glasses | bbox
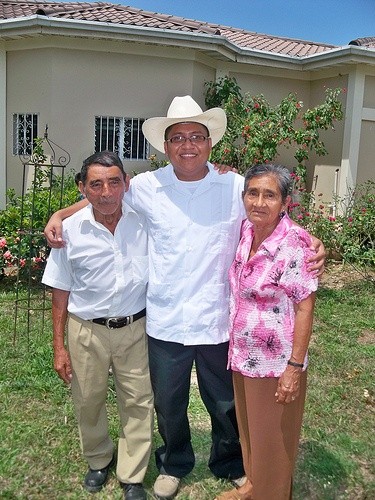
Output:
[166,135,209,144]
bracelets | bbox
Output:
[288,360,304,367]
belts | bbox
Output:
[92,308,148,329]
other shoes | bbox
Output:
[154,473,180,496]
[232,476,248,488]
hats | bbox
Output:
[142,96,228,154]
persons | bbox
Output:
[44,95,326,500]
[216,164,319,500]
[75,172,86,199]
[41,153,155,500]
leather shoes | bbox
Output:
[124,482,147,500]
[85,456,116,492]
